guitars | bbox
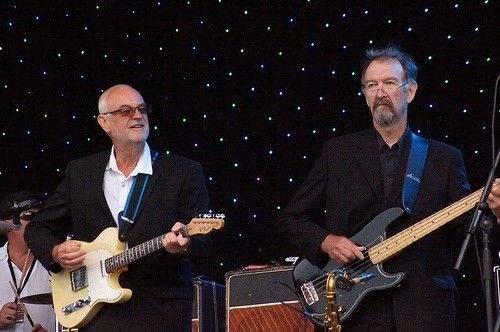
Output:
[293,178,500,326]
[51,213,225,329]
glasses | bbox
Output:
[360,81,408,97]
[103,102,153,117]
[6,211,36,221]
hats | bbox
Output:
[0,189,49,220]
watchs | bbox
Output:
[178,243,190,256]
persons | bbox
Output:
[0,190,56,332]
[277,47,500,332]
[24,84,210,332]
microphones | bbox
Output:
[11,201,22,230]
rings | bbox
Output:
[7,315,11,320]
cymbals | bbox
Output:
[19,292,52,305]
